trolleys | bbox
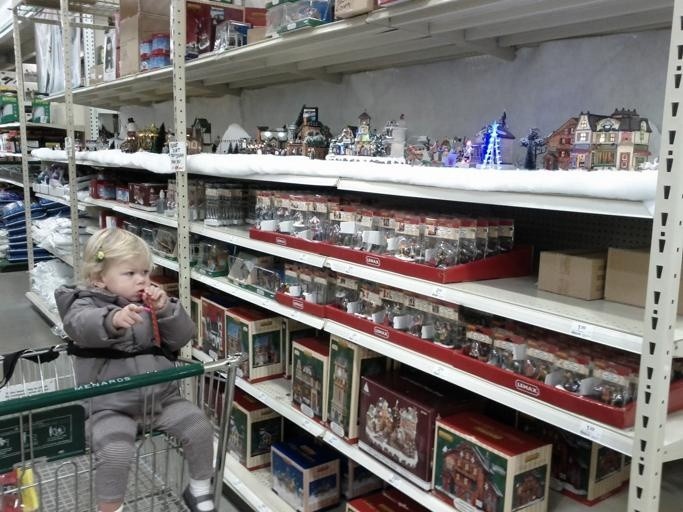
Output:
[0,340,250,511]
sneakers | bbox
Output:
[182,484,217,512]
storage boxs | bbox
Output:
[118,0,170,78]
[291,334,329,426]
[358,371,445,492]
[225,307,283,384]
[105,29,119,82]
[517,410,624,506]
[604,246,682,316]
[538,247,606,301]
[340,455,383,501]
[200,295,233,361]
[270,438,339,511]
[326,334,391,443]
[186,0,245,58]
[432,410,552,512]
[221,387,285,471]
[264,0,332,36]
[280,314,318,378]
[345,492,429,512]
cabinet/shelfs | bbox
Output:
[1,7,105,272]
[11,0,683,512]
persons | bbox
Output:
[53,226,219,512]
